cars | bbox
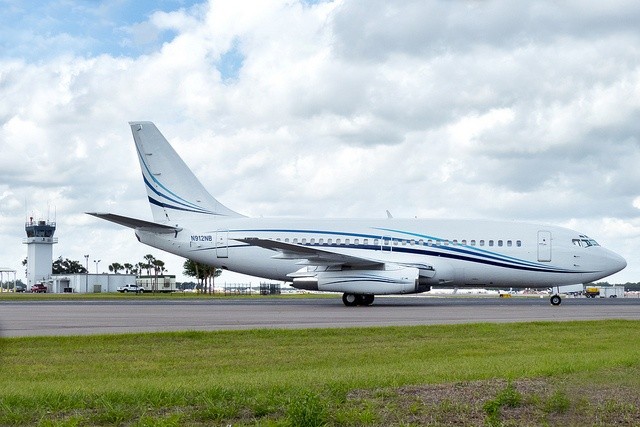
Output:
[11,286,25,293]
[117,284,145,293]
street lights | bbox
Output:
[94,259,101,274]
[85,254,89,292]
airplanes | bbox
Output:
[85,120,627,307]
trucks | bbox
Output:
[547,282,584,296]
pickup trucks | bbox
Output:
[31,283,47,293]
